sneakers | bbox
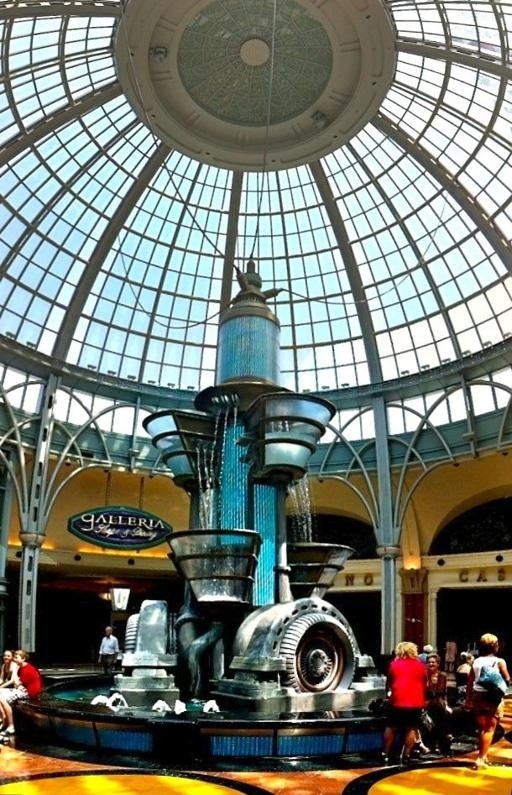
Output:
[471,756,492,770]
[381,744,432,766]
[0,722,15,738]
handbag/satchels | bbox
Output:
[476,665,508,699]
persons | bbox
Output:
[399,632,479,766]
[0,647,19,732]
[98,626,119,675]
[1,650,41,735]
[381,641,425,763]
[464,633,510,768]
